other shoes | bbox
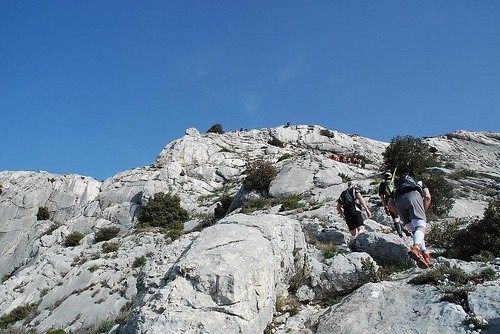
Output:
[403,228,411,236]
[400,235,403,238]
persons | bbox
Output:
[379,172,412,238]
[330,154,365,169]
[387,174,431,269]
[337,181,371,237]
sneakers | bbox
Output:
[422,250,430,263]
[408,247,428,269]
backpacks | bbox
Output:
[342,189,357,208]
[397,175,422,193]
[383,180,396,196]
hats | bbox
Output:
[385,172,392,179]
[348,181,356,185]
